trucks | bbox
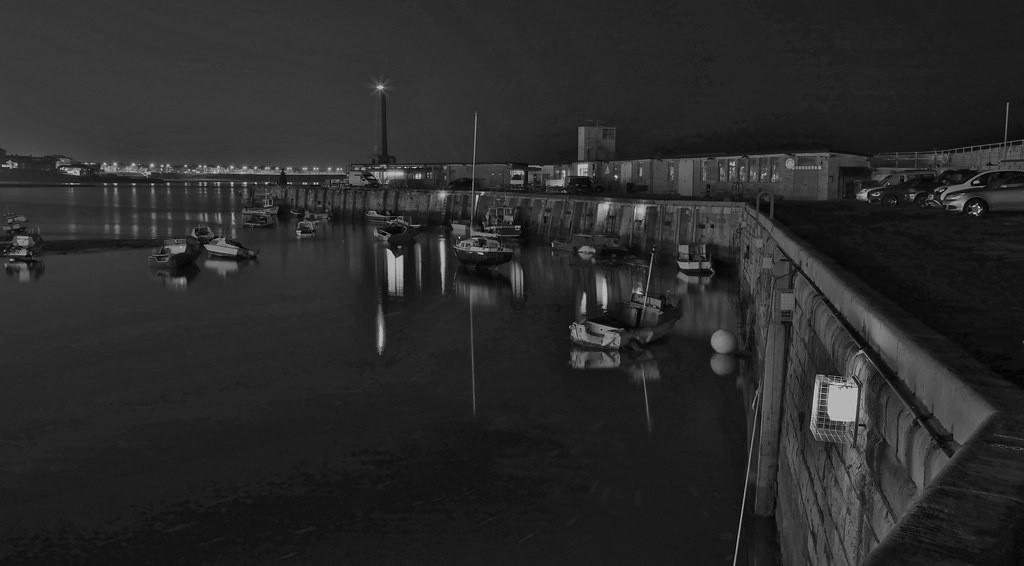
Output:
[349,171,379,188]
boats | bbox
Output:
[480,206,521,236]
[453,232,514,266]
[373,215,428,243]
[677,242,714,278]
[146,238,203,270]
[241,187,279,227]
[569,244,683,349]
[1,224,46,270]
[552,232,627,259]
[191,224,260,259]
[365,210,405,223]
[297,220,316,237]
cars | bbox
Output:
[856,168,1023,209]
[942,173,1024,220]
[564,175,605,194]
